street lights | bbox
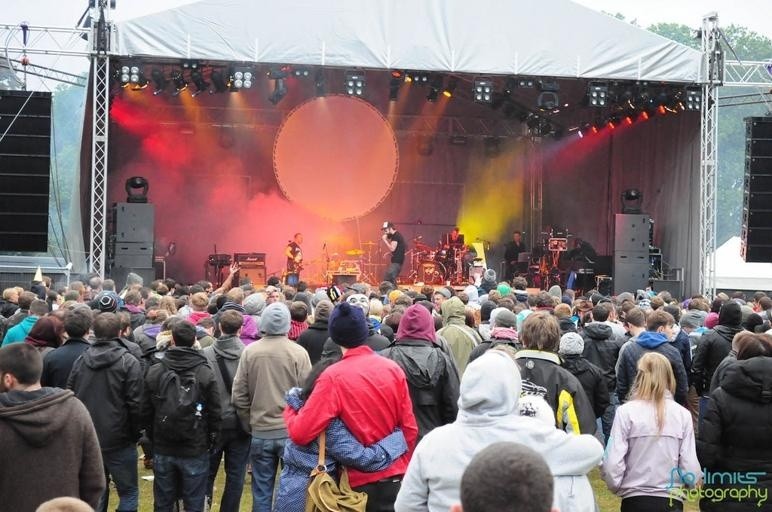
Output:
[304,464,369,512]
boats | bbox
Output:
[111,265,155,294]
[652,279,682,302]
[331,275,357,285]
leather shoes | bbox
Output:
[692,373,705,398]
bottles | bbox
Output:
[704,311,720,330]
[435,287,452,299]
[733,291,747,301]
[518,395,557,426]
[548,285,561,299]
[559,332,584,355]
[380,221,393,231]
[637,299,651,310]
[488,289,501,303]
[714,291,729,302]
[483,268,497,282]
[679,308,702,330]
[496,283,511,298]
[562,296,571,305]
[463,285,478,301]
[480,301,496,322]
[495,309,517,332]
[420,284,434,299]
[575,301,593,312]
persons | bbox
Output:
[447,227,464,244]
[284,233,304,286]
[570,238,598,264]
[504,231,527,273]
[382,221,406,283]
[461,243,477,273]
[0,260,772,512]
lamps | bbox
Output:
[113,58,701,141]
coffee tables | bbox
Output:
[359,243,378,245]
[346,250,366,254]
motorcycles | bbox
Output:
[360,245,380,286]
[407,252,421,280]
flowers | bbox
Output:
[323,241,326,249]
[476,237,489,243]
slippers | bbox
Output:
[152,362,204,450]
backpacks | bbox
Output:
[340,260,361,272]
[417,259,447,285]
